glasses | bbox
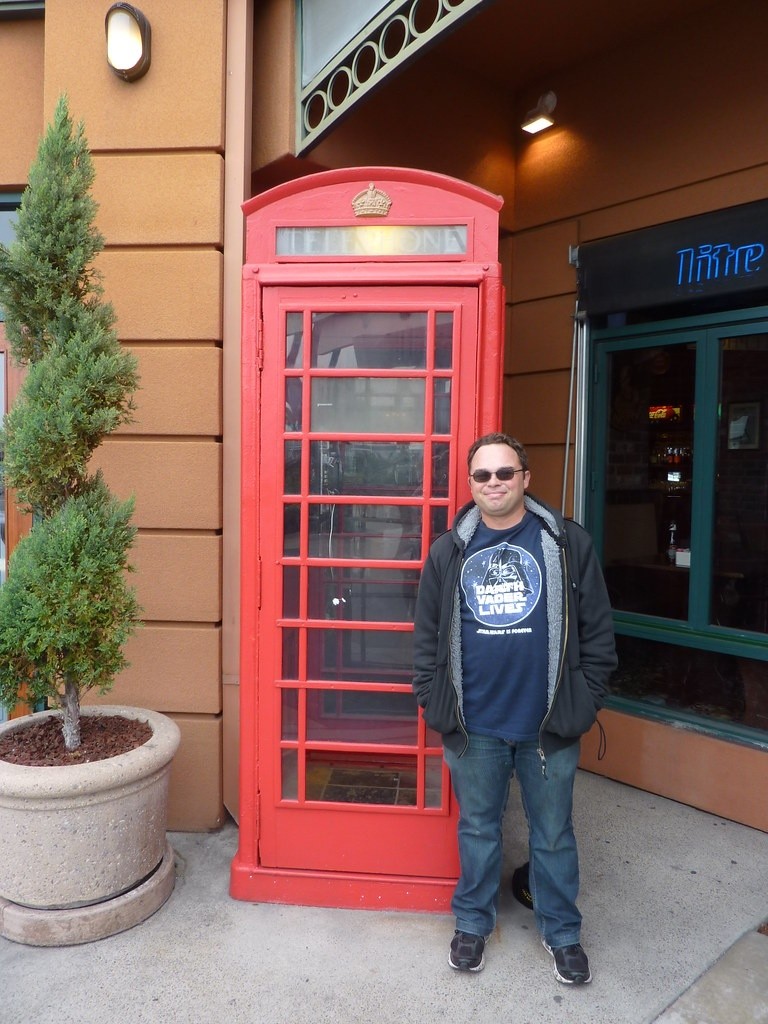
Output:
[469,467,528,482]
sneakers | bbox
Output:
[541,935,592,983]
[448,929,492,972]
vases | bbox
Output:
[726,399,762,452]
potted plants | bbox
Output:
[0,88,183,907]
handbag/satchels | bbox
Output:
[512,860,535,910]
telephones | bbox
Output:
[309,440,341,520]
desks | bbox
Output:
[623,554,768,620]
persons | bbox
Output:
[411,433,619,984]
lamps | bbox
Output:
[520,91,557,135]
[105,1,151,84]
